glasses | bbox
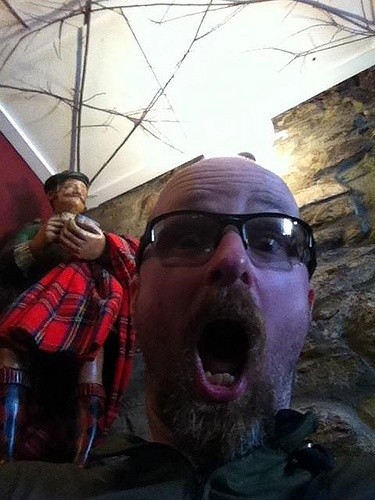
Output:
[134,208,318,281]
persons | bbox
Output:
[0,167,135,471]
[0,157,375,499]
